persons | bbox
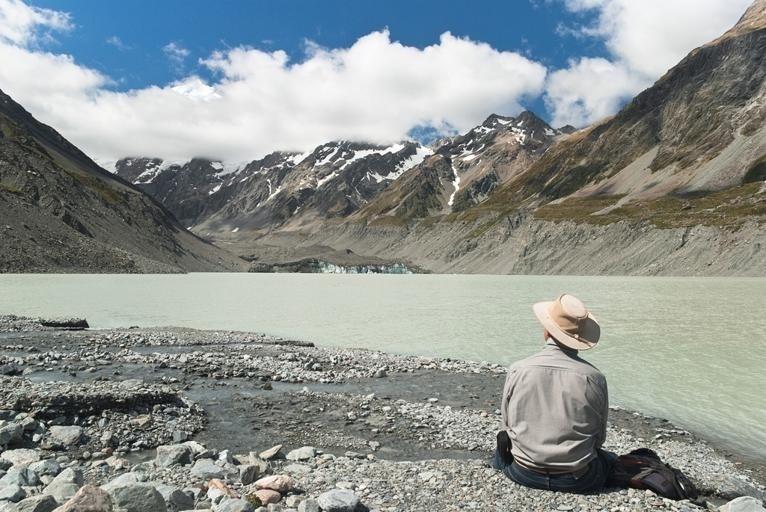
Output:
[495,293,607,490]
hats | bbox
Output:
[533,293,600,350]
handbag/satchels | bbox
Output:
[610,448,697,500]
